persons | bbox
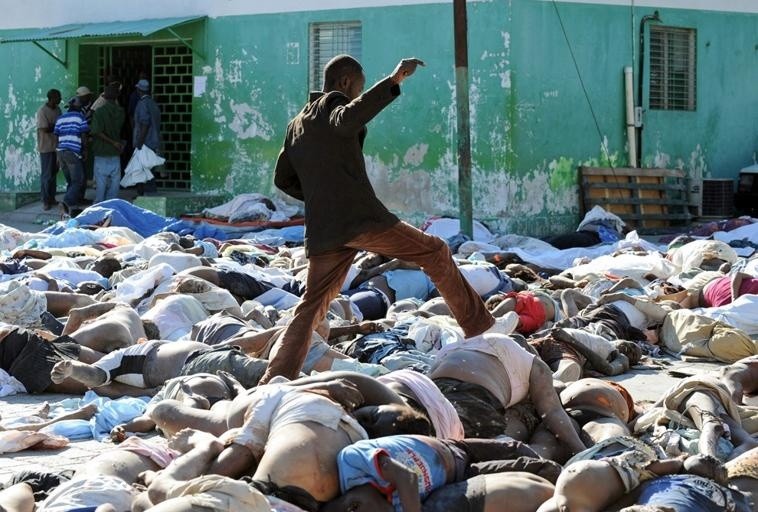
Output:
[0,188,758,511]
[36,69,164,215]
[251,52,519,387]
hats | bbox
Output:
[68,75,150,106]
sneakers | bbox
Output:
[478,309,519,338]
[39,196,89,216]
